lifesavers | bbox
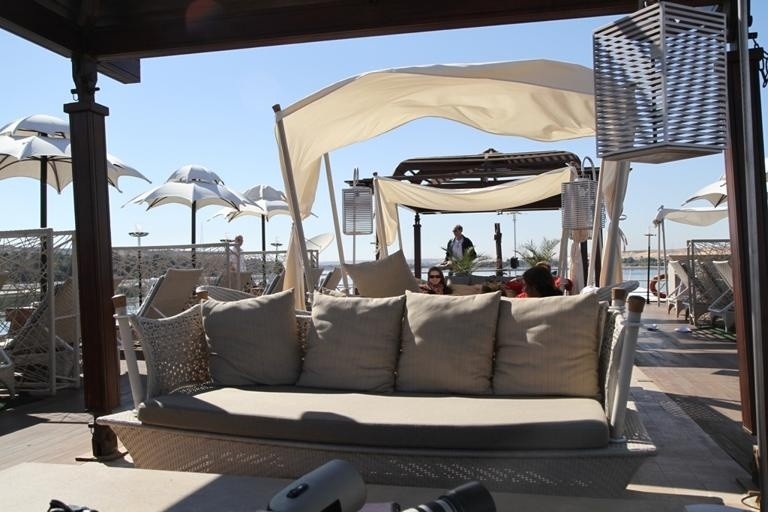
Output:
[650,275,666,298]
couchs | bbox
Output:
[90,286,657,497]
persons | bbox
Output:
[520,268,563,296]
[532,260,552,275]
[440,223,477,277]
[416,265,454,295]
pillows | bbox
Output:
[341,249,422,296]
[391,288,503,395]
[493,290,599,398]
[295,288,404,394]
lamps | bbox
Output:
[341,167,372,263]
[563,155,599,243]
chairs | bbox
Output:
[1,263,84,404]
[113,263,203,351]
[708,257,734,331]
[202,263,341,309]
[664,261,704,317]
[682,261,728,326]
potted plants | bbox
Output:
[437,244,485,286]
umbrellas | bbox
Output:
[681,178,729,210]
[0,111,153,304]
[205,183,317,282]
[120,166,262,267]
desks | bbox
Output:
[0,464,728,511]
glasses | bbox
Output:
[429,275,441,278]
